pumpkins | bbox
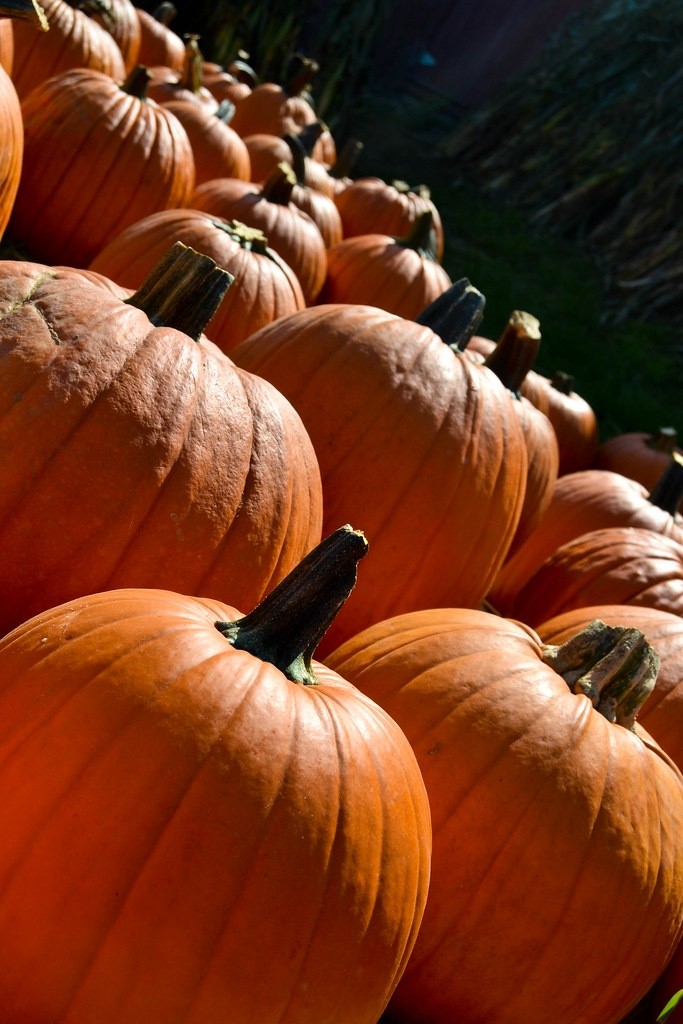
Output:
[0,0,682,1024]
